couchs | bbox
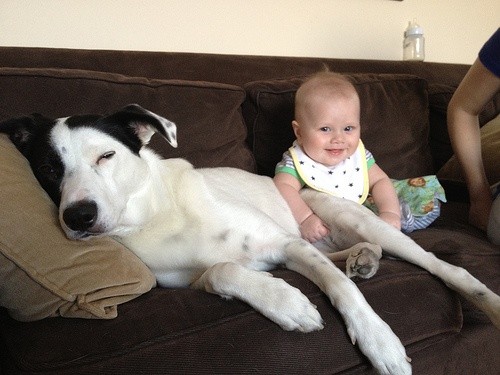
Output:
[0,47,500,375]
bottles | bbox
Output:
[403,18,425,60]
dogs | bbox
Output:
[2,103,500,375]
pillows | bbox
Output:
[0,133,157,320]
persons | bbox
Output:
[447,25,500,247]
[274,71,447,243]
